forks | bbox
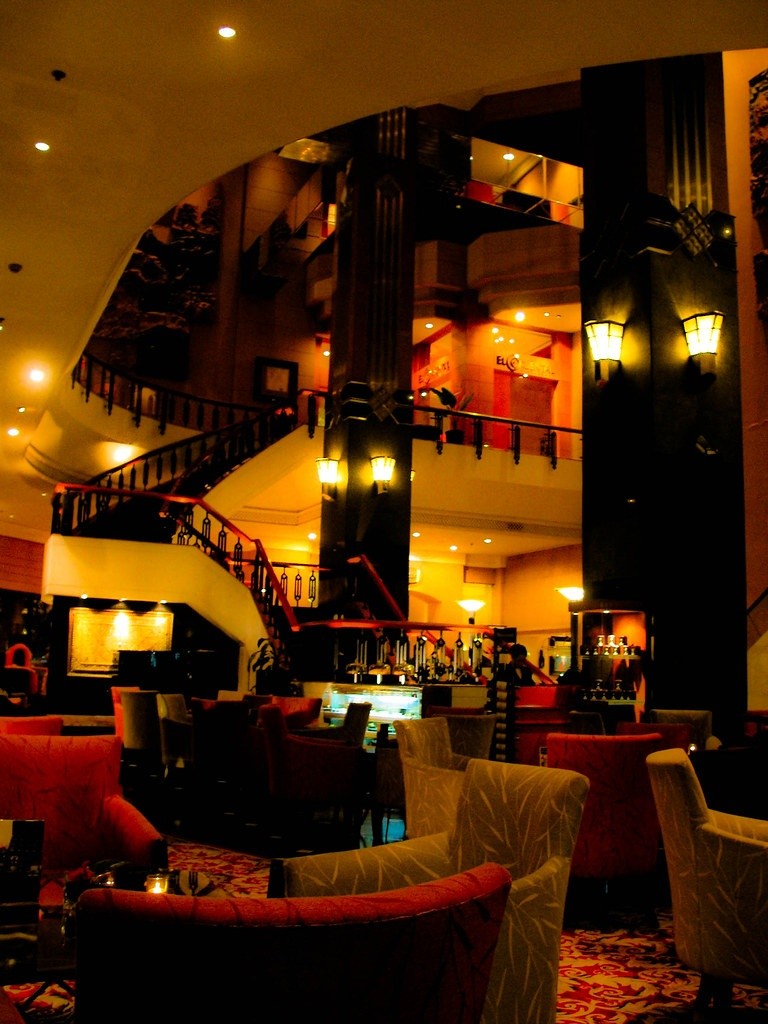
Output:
[189,871,198,896]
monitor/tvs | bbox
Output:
[329,684,424,741]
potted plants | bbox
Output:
[430,387,473,445]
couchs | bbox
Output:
[0,686,768,1024]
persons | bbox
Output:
[504,645,536,688]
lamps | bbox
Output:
[370,456,396,495]
[420,389,428,397]
[584,320,626,381]
[681,311,726,385]
[315,458,339,502]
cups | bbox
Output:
[147,875,169,893]
